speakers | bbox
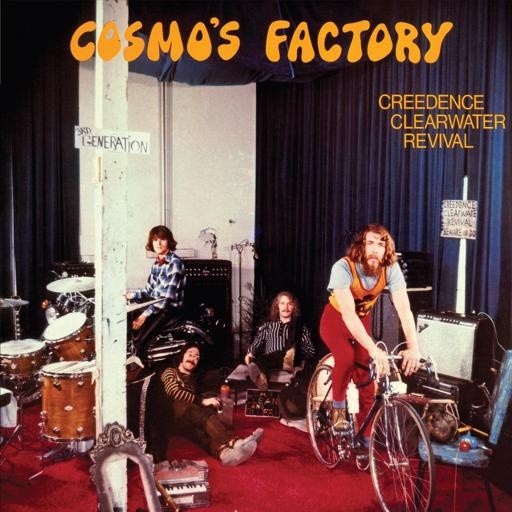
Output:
[372,287,495,427]
[180,259,234,373]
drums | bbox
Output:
[0,339,46,381]
[41,311,95,362]
[38,360,96,444]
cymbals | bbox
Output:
[0,297,28,311]
[46,274,95,294]
[125,297,169,313]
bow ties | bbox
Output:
[153,256,166,266]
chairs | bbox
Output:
[414,350,512,512]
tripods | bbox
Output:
[0,383,51,469]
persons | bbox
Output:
[153,344,266,468]
[318,222,422,480]
[245,287,317,392]
[123,225,188,369]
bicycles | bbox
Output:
[305,343,435,511]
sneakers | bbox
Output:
[248,362,268,391]
[222,428,263,466]
[332,410,350,432]
[283,350,296,374]
[356,444,370,471]
